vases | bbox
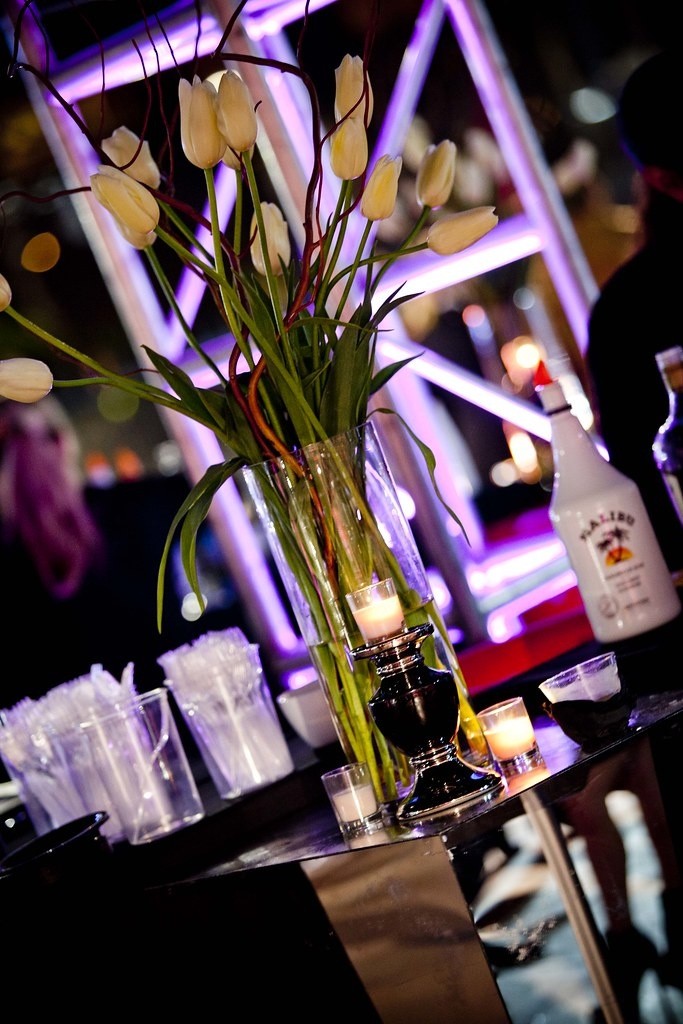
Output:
[242,422,506,828]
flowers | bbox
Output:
[0,0,499,803]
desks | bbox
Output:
[145,681,683,1024]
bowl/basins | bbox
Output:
[0,811,109,882]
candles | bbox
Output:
[345,577,403,641]
[476,696,542,779]
[321,762,387,844]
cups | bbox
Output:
[476,698,539,771]
[0,723,87,835]
[163,642,295,802]
[278,676,337,748]
[39,692,138,845]
[322,763,385,841]
[540,652,632,753]
[346,579,408,646]
[84,690,206,847]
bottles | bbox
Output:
[535,380,680,645]
[654,346,683,526]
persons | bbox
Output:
[583,46,683,850]
